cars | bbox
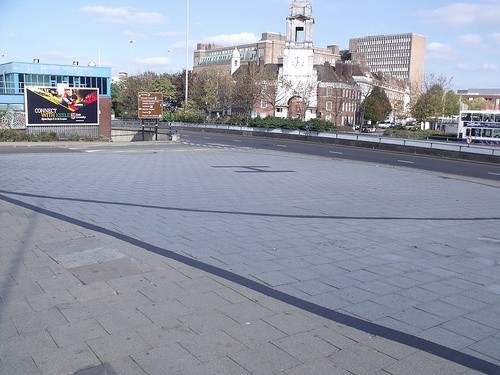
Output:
[353,119,422,138]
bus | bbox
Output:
[458,109,500,146]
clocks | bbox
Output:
[290,55,306,70]
